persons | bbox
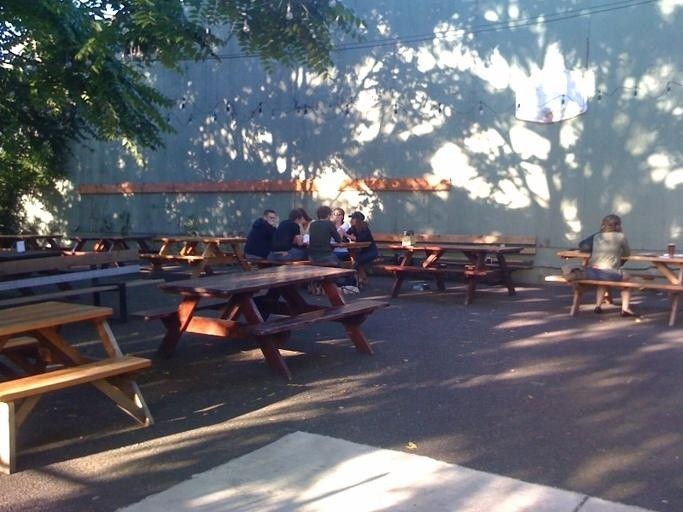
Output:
[308,205,342,296]
[265,209,308,262]
[586,214,639,317]
[340,211,378,289]
[242,209,276,270]
[297,207,315,245]
[329,207,350,261]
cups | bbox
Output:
[667,243,676,259]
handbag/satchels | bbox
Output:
[560,263,587,280]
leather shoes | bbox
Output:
[621,310,639,317]
[594,306,601,312]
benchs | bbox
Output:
[554,250,683,326]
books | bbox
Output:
[663,252,682,258]
[637,250,659,257]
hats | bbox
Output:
[349,212,364,220]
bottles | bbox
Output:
[399,230,416,247]
